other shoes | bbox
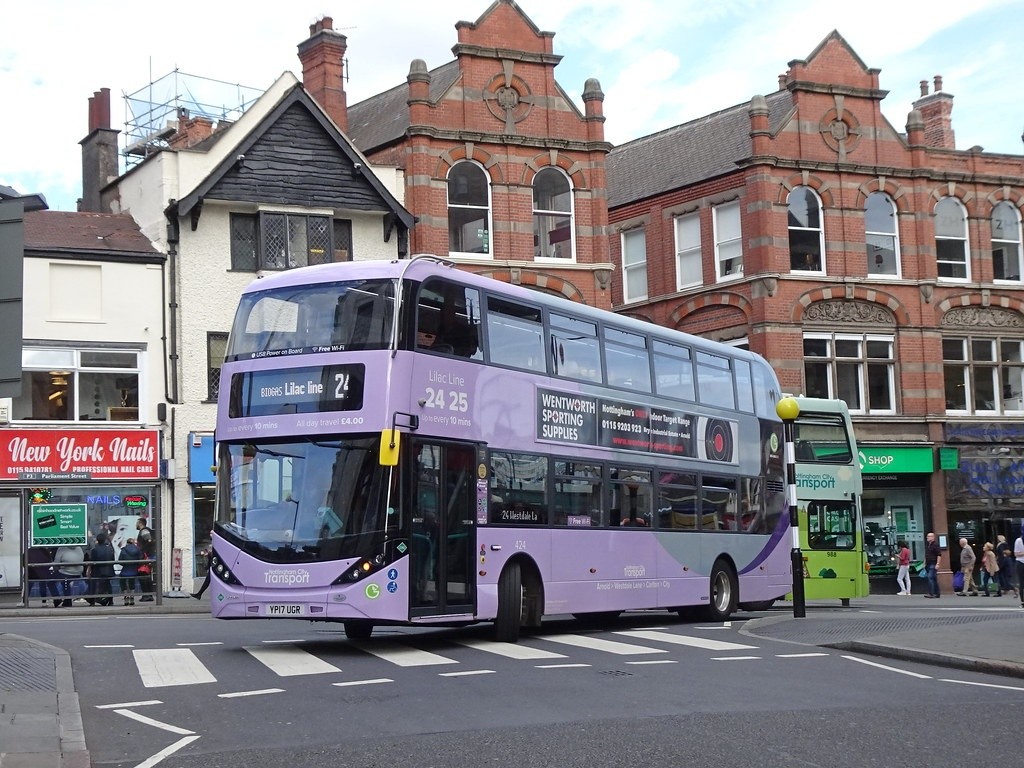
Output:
[16,602,29,608]
[897,591,911,596]
[62,599,72,608]
[140,595,154,602]
[41,603,49,608]
[981,595,990,597]
[190,594,201,601]
[54,599,62,608]
[102,599,114,606]
[924,594,940,598]
[1002,591,1010,595]
[1013,587,1019,598]
[124,597,134,605]
[993,594,1001,597]
[956,592,967,596]
[969,592,978,596]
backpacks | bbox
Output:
[140,527,156,557]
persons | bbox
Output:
[189,530,212,600]
[22,518,156,607]
[894,541,911,596]
[956,538,978,596]
[923,533,942,598]
[980,535,1019,598]
[1014,526,1024,609]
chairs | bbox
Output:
[720,511,761,530]
[453,337,475,356]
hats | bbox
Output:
[997,535,1005,542]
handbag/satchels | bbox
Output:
[919,566,928,577]
[952,571,964,589]
[987,576,998,592]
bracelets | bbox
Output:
[937,563,940,565]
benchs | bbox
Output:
[29,575,155,605]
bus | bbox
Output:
[781,396,870,601]
[207,253,793,643]
